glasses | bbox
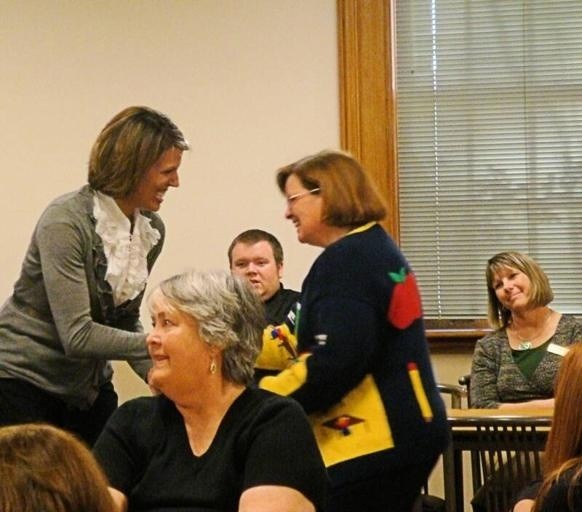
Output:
[286,187,320,209]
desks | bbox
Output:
[430,405,560,512]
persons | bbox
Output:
[227,229,302,336]
[503,336,582,512]
[250,149,449,512]
[0,422,119,512]
[0,105,189,448]
[87,267,334,512]
[468,250,581,512]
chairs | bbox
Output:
[437,414,556,511]
[457,371,493,512]
[419,380,469,512]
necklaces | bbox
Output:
[511,307,554,341]
